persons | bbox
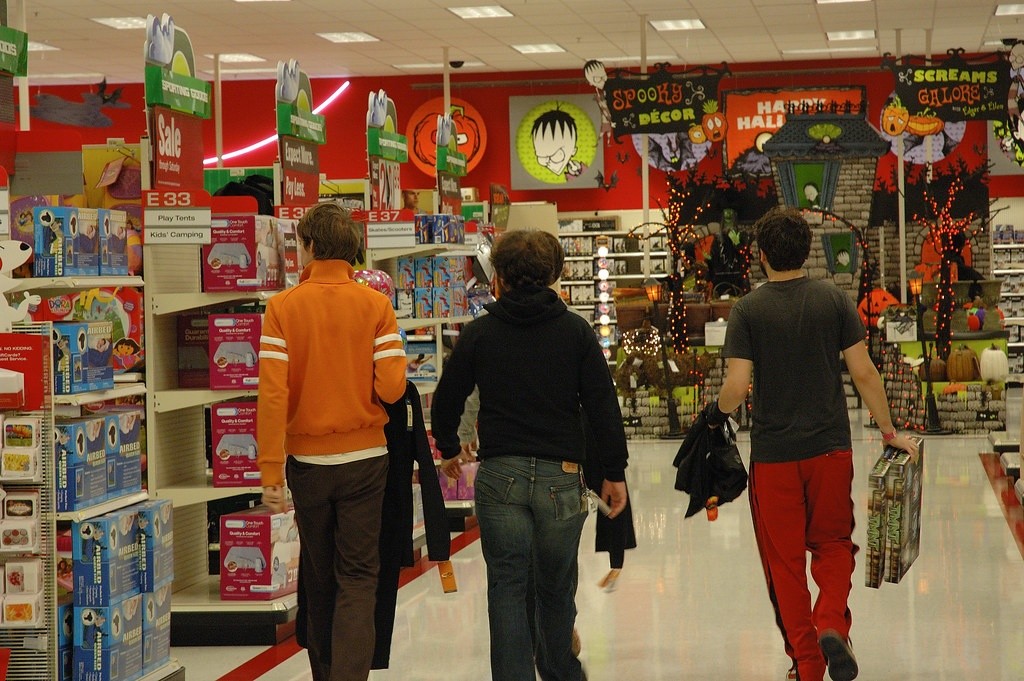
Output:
[256,204,406,681]
[705,205,919,681]
[429,230,626,681]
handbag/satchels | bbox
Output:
[707,408,748,502]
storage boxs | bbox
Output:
[864,435,925,590]
[0,205,675,681]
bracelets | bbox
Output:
[883,429,896,439]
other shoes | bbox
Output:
[821,629,858,681]
[786,668,797,681]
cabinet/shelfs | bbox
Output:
[555,230,669,366]
[990,244,1024,388]
[364,244,482,532]
[0,275,187,681]
[144,290,300,646]
